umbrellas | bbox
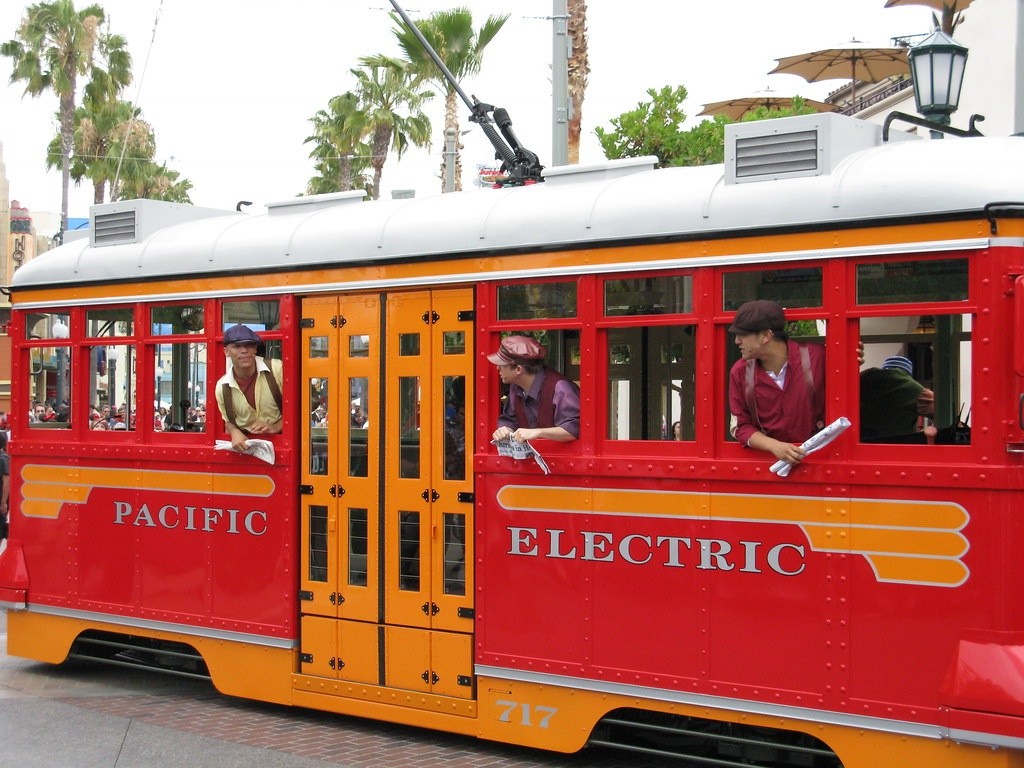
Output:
[767,0,977,110]
[695,85,845,122]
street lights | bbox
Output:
[903,15,970,440]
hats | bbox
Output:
[222,325,262,345]
[882,355,913,376]
[727,299,785,334]
[486,335,546,367]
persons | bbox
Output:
[0,295,947,565]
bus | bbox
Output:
[1,0,1024,768]
